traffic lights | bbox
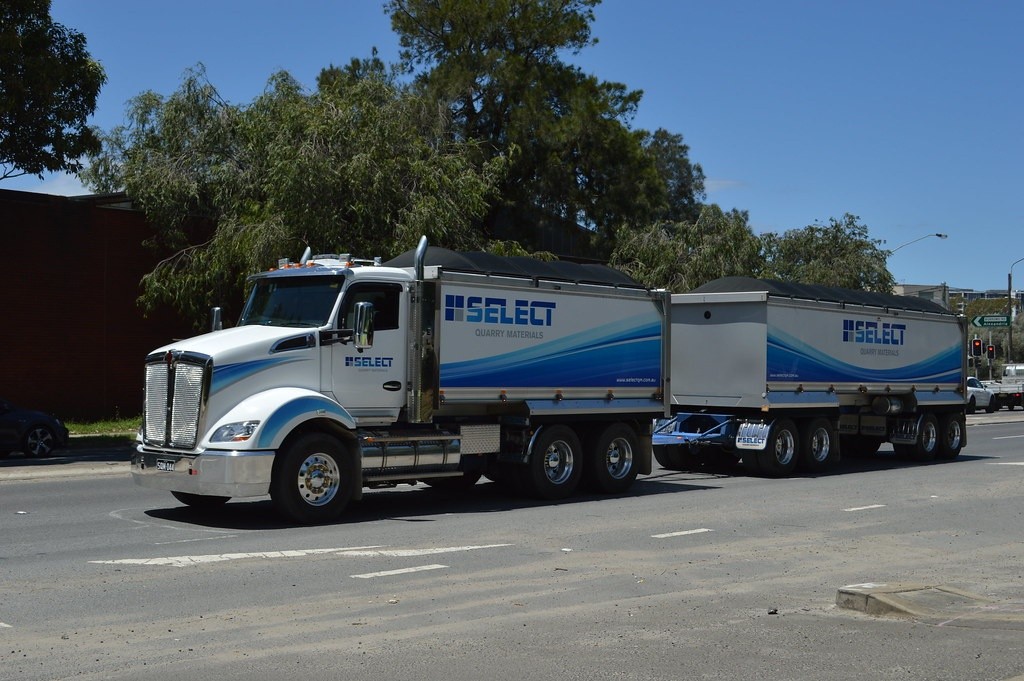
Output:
[971,338,983,356]
[973,356,982,368]
[987,344,996,360]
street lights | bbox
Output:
[880,232,949,292]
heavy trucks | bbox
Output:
[128,231,969,523]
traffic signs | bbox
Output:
[972,314,1010,327]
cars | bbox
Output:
[966,376,997,414]
[0,395,70,461]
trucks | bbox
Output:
[979,363,1024,410]
